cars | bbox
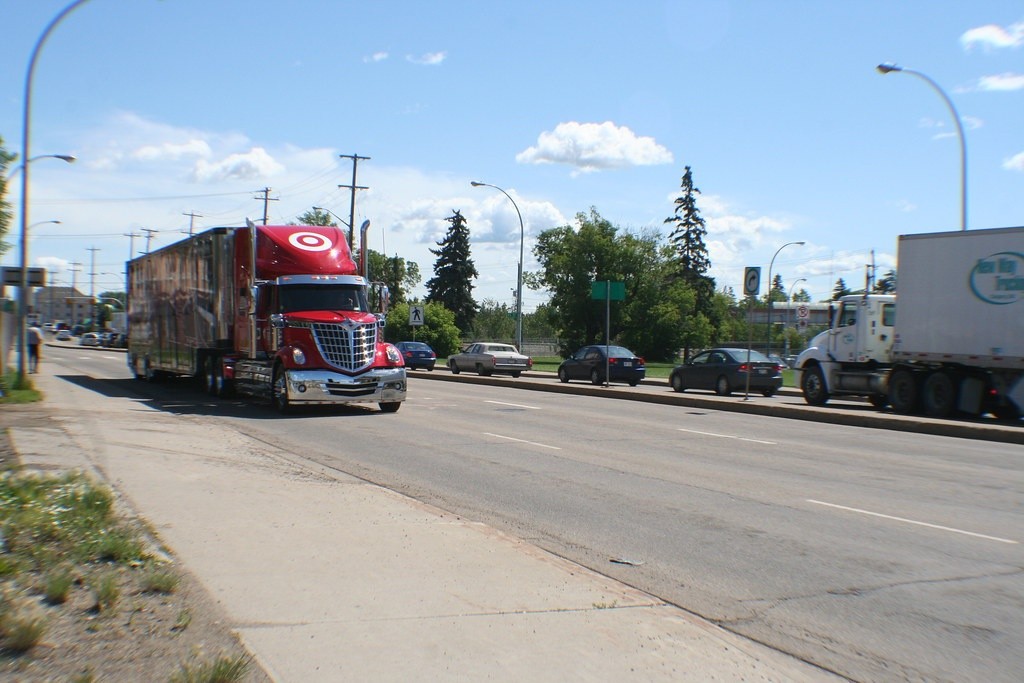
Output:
[445,342,533,378]
[558,345,647,387]
[43,320,128,348]
[669,347,789,399]
[394,341,438,372]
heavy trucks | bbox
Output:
[123,224,408,417]
[794,226,1024,430]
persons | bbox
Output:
[28,322,43,373]
[337,290,353,306]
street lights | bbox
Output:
[877,62,968,231]
[313,205,353,251]
[785,278,808,352]
[765,240,805,356]
[472,181,524,356]
